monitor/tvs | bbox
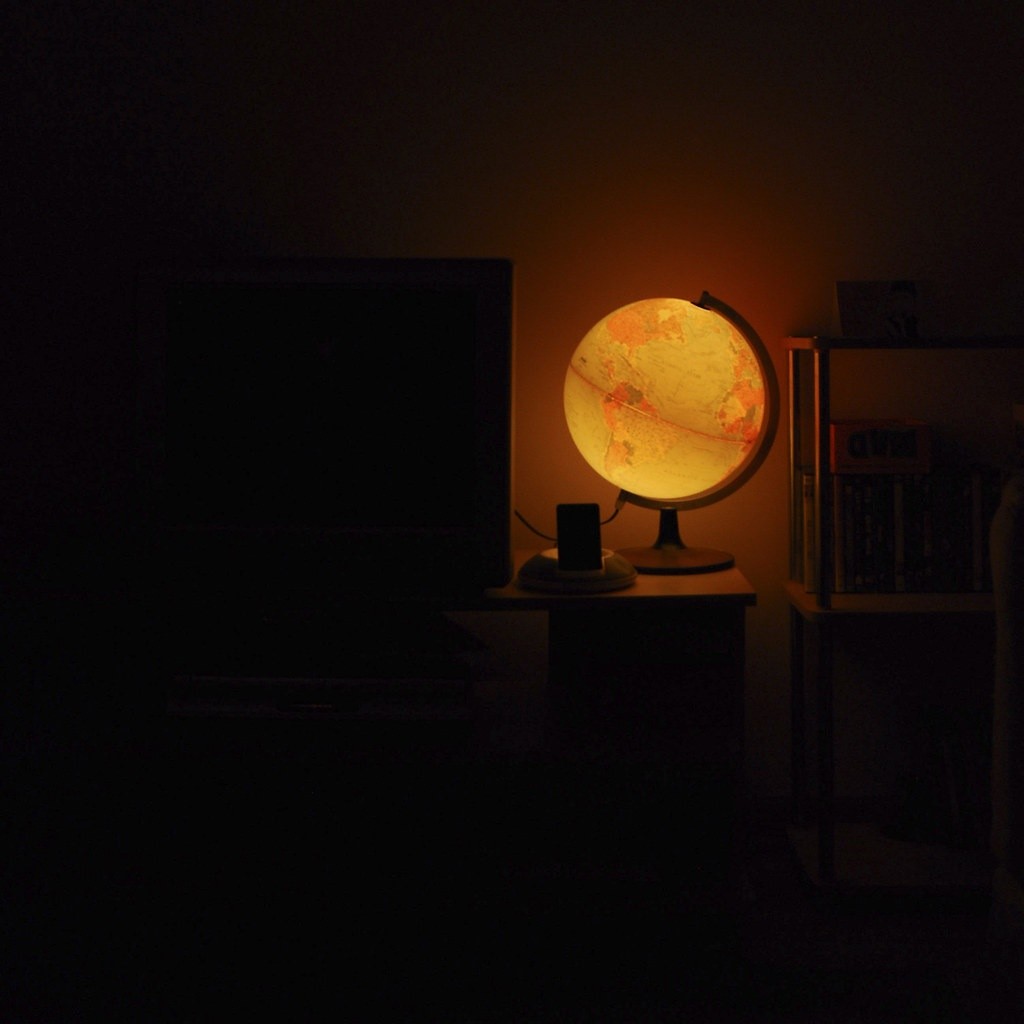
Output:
[134,258,512,598]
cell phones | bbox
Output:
[557,504,601,572]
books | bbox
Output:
[800,475,1005,593]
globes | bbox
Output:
[564,291,780,575]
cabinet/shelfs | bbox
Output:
[98,551,764,892]
[789,336,1024,914]
